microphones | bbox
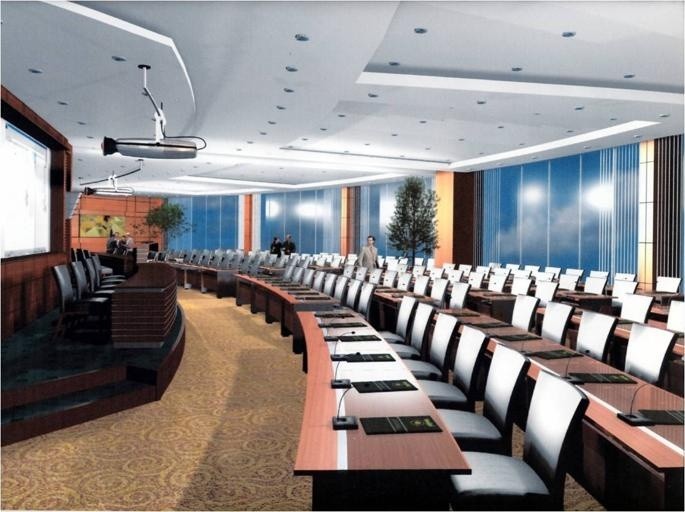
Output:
[331,330,358,362]
[331,382,372,429]
[331,352,362,389]
[323,313,345,341]
[518,325,538,358]
[559,349,592,384]
[618,376,672,426]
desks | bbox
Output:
[295,310,471,510]
[432,312,684,508]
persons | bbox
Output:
[358,236,380,275]
[282,234,296,255]
[106,232,134,256]
[269,237,283,258]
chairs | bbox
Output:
[378,296,417,343]
[449,370,587,510]
[52,250,120,355]
[390,302,435,360]
[416,323,490,407]
[434,345,530,455]
[145,247,375,327]
[511,293,685,387]
[400,312,461,380]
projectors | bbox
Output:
[84,186,134,197]
[102,134,198,159]
